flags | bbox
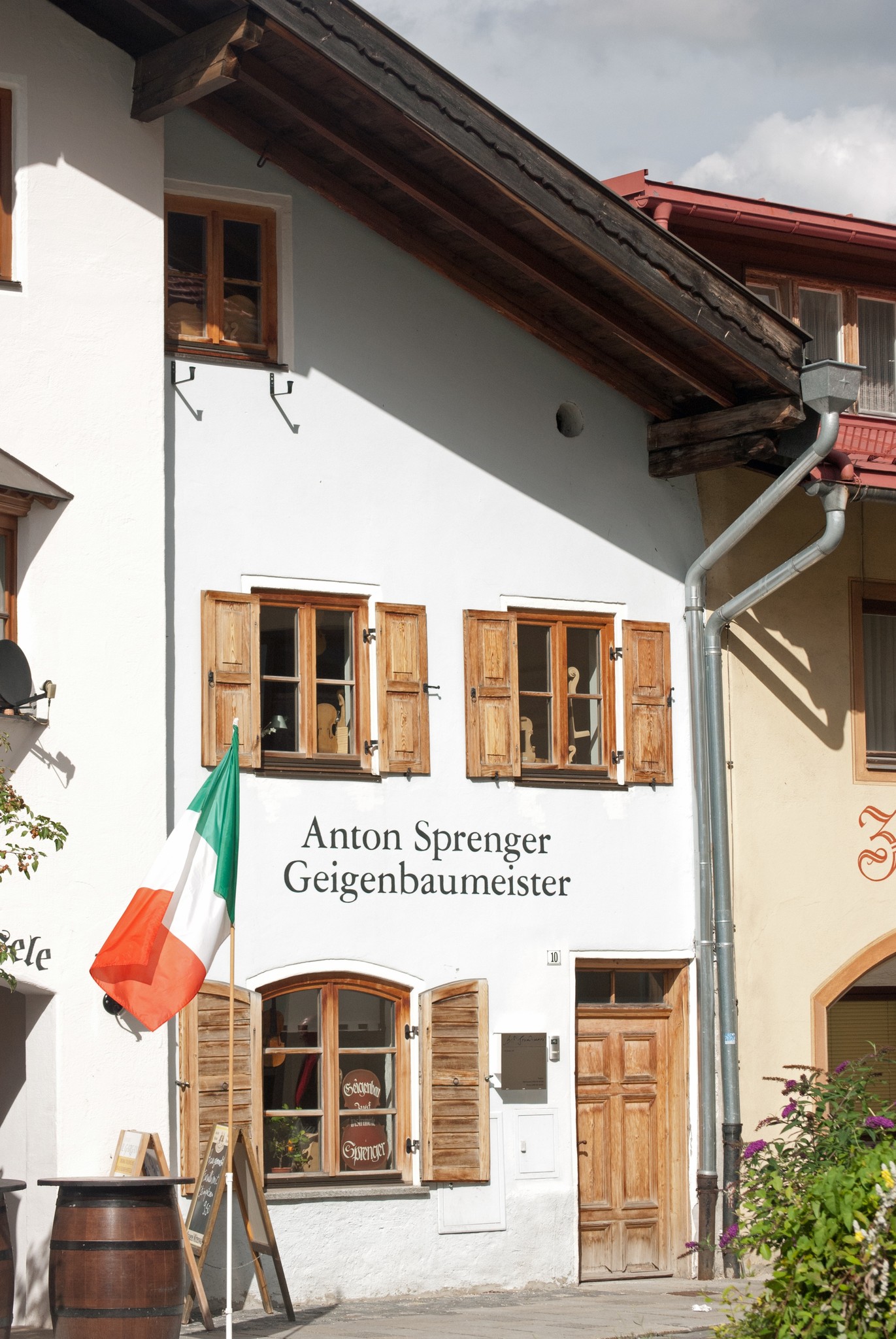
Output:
[90,727,240,1033]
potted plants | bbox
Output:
[264,1103,310,1172]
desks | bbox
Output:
[37,1176,195,1339]
[0,1178,26,1339]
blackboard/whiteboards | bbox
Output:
[110,1129,188,1239]
[185,1122,276,1257]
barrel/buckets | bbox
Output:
[0,1192,15,1339]
[49,1186,186,1339]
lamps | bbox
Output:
[263,715,288,730]
